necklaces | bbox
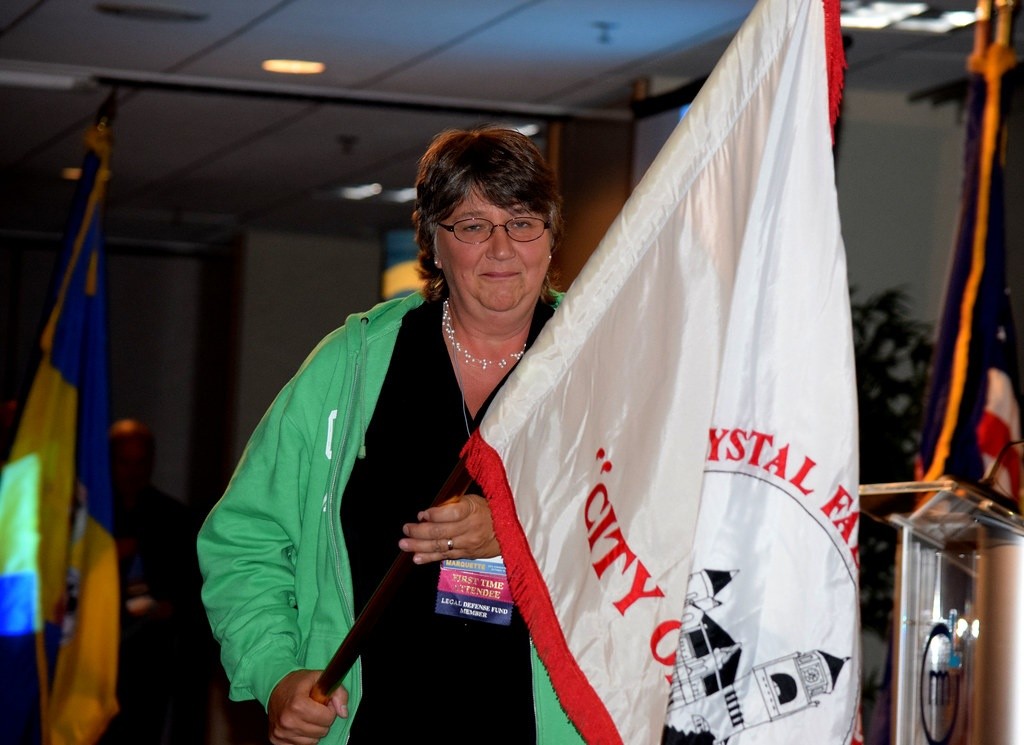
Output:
[437,293,532,374]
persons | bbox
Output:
[193,123,592,745]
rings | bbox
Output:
[447,536,455,550]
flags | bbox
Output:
[456,0,873,745]
[0,98,130,745]
[909,2,1023,549]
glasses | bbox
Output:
[431,216,553,246]
